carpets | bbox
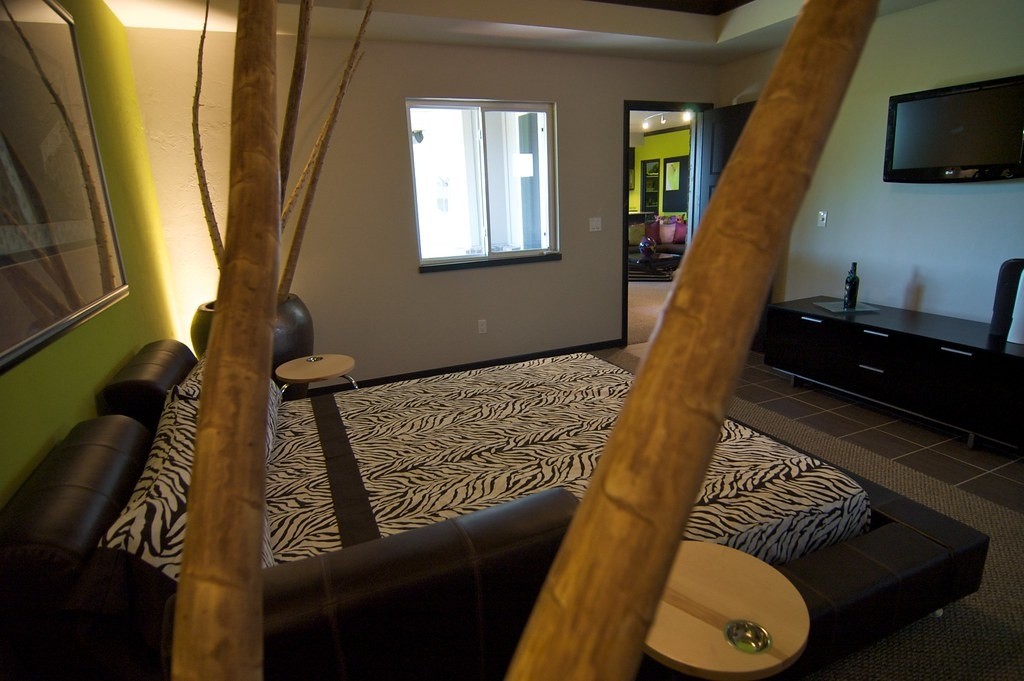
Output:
[593,344,1024,681]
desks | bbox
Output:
[275,354,359,394]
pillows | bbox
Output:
[107,401,271,589]
[174,347,284,430]
[629,214,688,245]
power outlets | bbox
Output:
[478,319,488,334]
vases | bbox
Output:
[189,293,315,399]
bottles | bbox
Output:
[843,262,860,307]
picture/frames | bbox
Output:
[0,0,129,373]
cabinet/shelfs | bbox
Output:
[763,296,1024,455]
[646,175,658,209]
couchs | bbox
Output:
[629,221,687,255]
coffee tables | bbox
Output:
[628,253,680,281]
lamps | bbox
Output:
[660,114,667,124]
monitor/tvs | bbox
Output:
[884,74,1024,184]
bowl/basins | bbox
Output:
[723,619,773,655]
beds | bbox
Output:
[15,337,991,665]
